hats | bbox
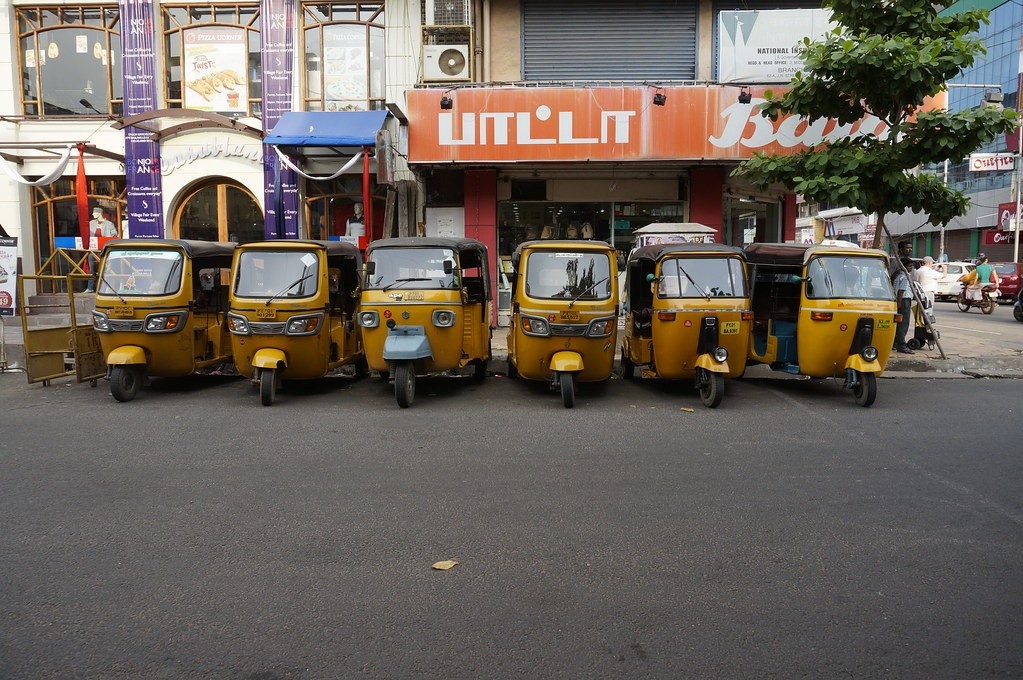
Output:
[923,256,935,263]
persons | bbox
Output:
[889,240,916,355]
[960,252,1002,305]
[81,207,118,295]
[344,202,367,265]
[911,255,948,331]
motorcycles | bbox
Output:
[743,242,902,409]
[359,236,488,408]
[93,240,239,404]
[228,239,369,404]
[957,283,996,315]
[624,243,757,409]
[505,240,628,407]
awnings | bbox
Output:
[262,109,394,262]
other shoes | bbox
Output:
[898,347,915,355]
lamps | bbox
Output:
[737,91,752,105]
[653,91,668,107]
[440,93,454,111]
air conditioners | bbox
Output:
[422,44,468,78]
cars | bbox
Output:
[985,261,1023,306]
[889,257,935,286]
[928,261,977,303]
[964,258,982,267]
